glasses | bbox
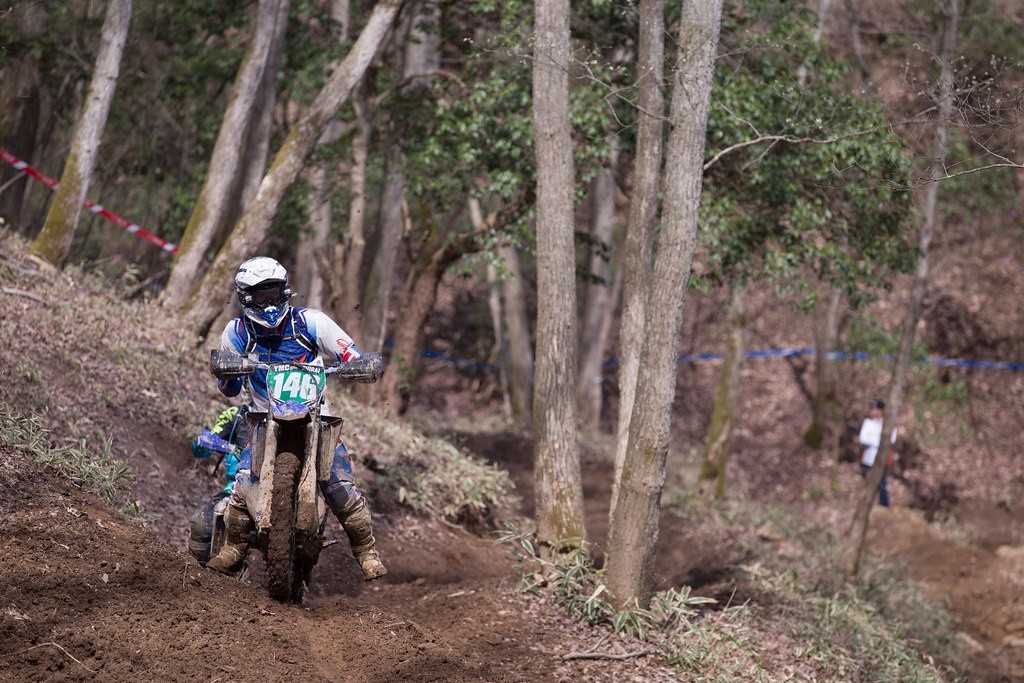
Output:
[248,283,288,308]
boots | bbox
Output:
[336,496,387,581]
[206,499,255,574]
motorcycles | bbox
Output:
[196,360,381,604]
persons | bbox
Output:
[859,400,898,507]
[205,256,387,583]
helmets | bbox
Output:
[234,256,298,328]
